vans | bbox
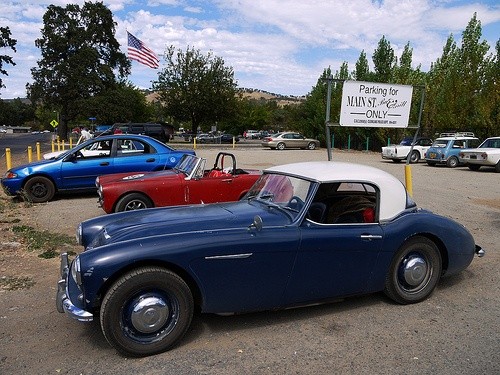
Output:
[98,121,166,143]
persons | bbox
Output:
[80,127,124,147]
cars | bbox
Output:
[261,131,321,150]
[197,133,239,144]
[32,130,51,133]
[0,131,197,204]
[54,159,485,358]
[459,137,500,172]
[424,131,481,168]
[94,151,293,214]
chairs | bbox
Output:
[209,170,232,177]
[310,203,327,224]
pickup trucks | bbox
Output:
[381,137,459,163]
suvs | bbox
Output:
[243,129,269,139]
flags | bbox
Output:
[126,31,159,69]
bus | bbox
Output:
[7,125,32,133]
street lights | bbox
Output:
[53,109,59,126]
